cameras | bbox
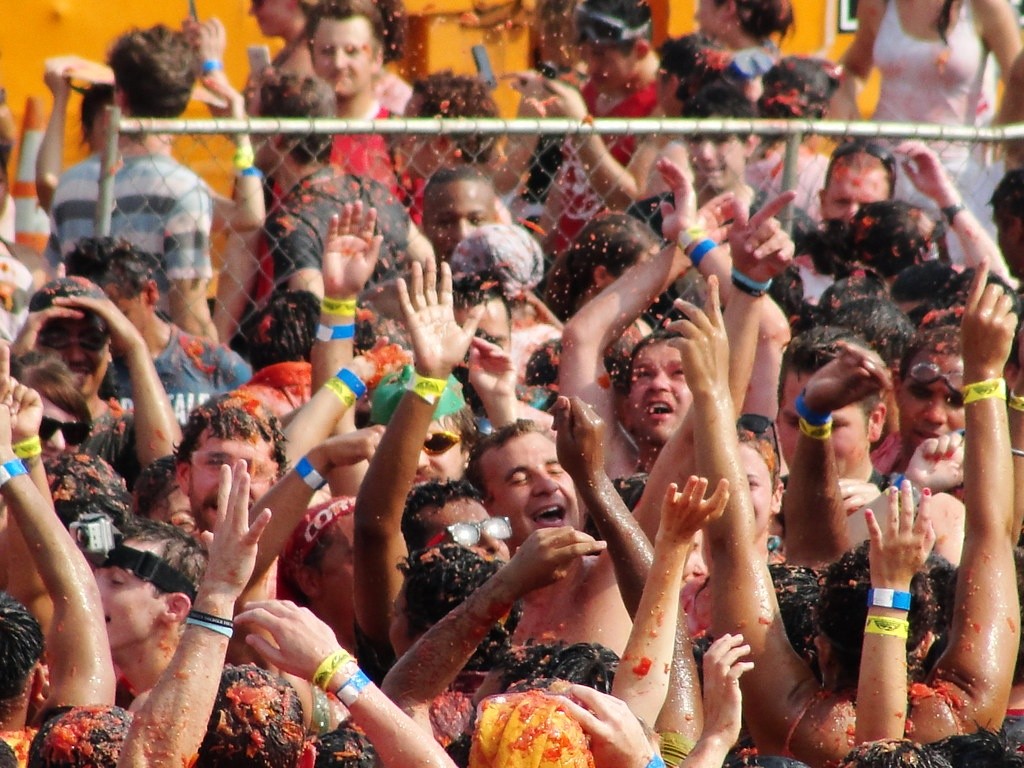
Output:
[68,514,114,556]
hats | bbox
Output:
[27,276,106,331]
[519,339,560,407]
[371,367,464,425]
[246,363,312,389]
[759,58,830,113]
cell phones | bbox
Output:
[247,46,270,71]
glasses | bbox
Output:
[424,517,513,547]
[832,143,895,173]
[39,328,109,350]
[904,363,966,394]
[422,433,462,453]
[575,6,651,44]
[737,414,781,469]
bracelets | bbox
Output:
[799,417,832,440]
[795,390,831,426]
[316,322,354,341]
[960,378,1009,403]
[689,239,716,268]
[324,376,356,408]
[864,614,912,639]
[311,648,358,691]
[293,456,328,491]
[320,297,357,315]
[232,149,253,167]
[408,373,449,404]
[235,167,263,180]
[884,473,926,518]
[730,265,773,297]
[336,368,366,398]
[200,59,224,73]
[676,223,706,253]
[184,608,235,638]
[1006,393,1024,412]
[867,588,913,610]
[335,669,371,708]
[0,457,27,489]
[11,435,42,460]
[641,751,667,768]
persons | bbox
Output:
[0,0,1024,768]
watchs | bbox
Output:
[939,202,970,226]
[768,535,781,551]
